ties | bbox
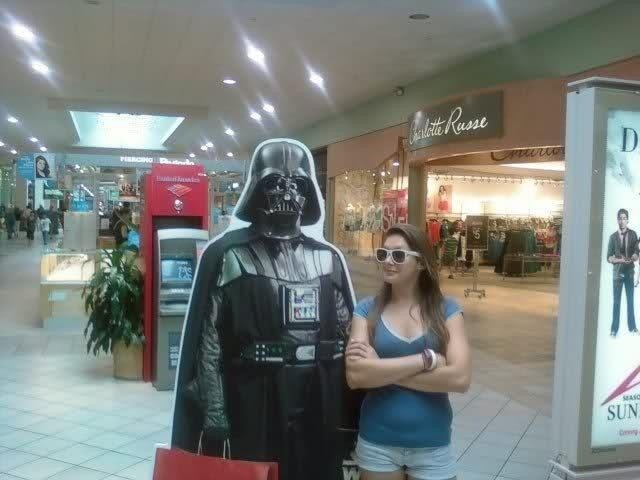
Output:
[622,233,627,258]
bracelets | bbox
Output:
[422,347,437,371]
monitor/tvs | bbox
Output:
[68,197,96,211]
[160,258,194,281]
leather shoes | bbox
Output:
[611,328,618,338]
[629,327,639,335]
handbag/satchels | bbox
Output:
[151,444,277,480]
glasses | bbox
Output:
[375,247,421,264]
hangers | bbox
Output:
[430,218,437,222]
[441,218,449,223]
[455,217,464,223]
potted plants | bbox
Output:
[80,235,144,380]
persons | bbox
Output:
[167,136,357,479]
[0,203,65,245]
[113,203,131,247]
[35,155,52,178]
[608,209,639,338]
[345,223,473,480]
[438,185,449,210]
